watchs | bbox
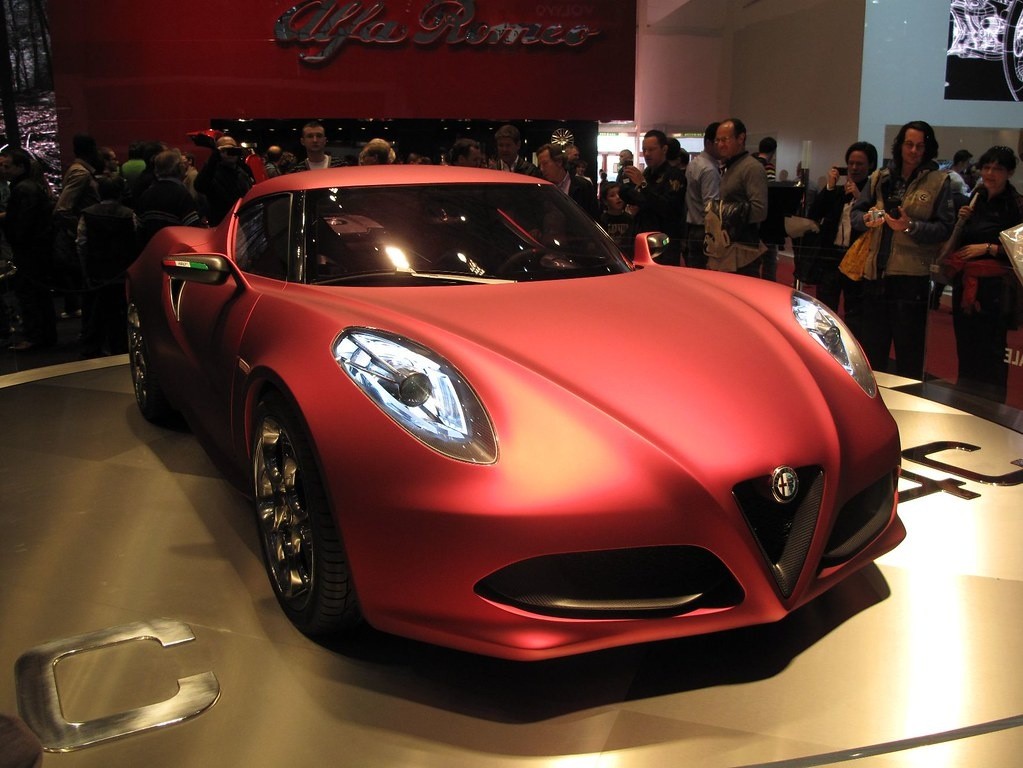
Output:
[638,180,647,189]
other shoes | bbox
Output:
[57,308,81,320]
[8,340,39,353]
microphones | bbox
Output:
[958,184,986,227]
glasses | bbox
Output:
[714,134,737,143]
[902,140,926,152]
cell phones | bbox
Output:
[623,159,634,168]
[833,165,849,177]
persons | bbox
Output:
[196,137,254,226]
[446,139,483,169]
[360,143,390,164]
[136,151,207,254]
[74,173,137,358]
[53,133,168,317]
[1,149,47,351]
[180,154,199,199]
[810,142,877,341]
[535,143,598,224]
[290,122,350,174]
[753,137,778,280]
[563,144,635,181]
[624,129,687,266]
[682,122,723,268]
[665,137,690,177]
[262,145,285,178]
[598,181,631,255]
[848,121,954,382]
[947,148,974,174]
[489,125,542,179]
[714,119,769,278]
[944,146,1023,405]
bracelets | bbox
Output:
[986,243,991,255]
[904,218,914,235]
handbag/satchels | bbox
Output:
[961,259,1023,331]
[840,226,876,281]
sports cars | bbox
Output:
[119,160,907,671]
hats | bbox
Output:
[216,136,237,150]
